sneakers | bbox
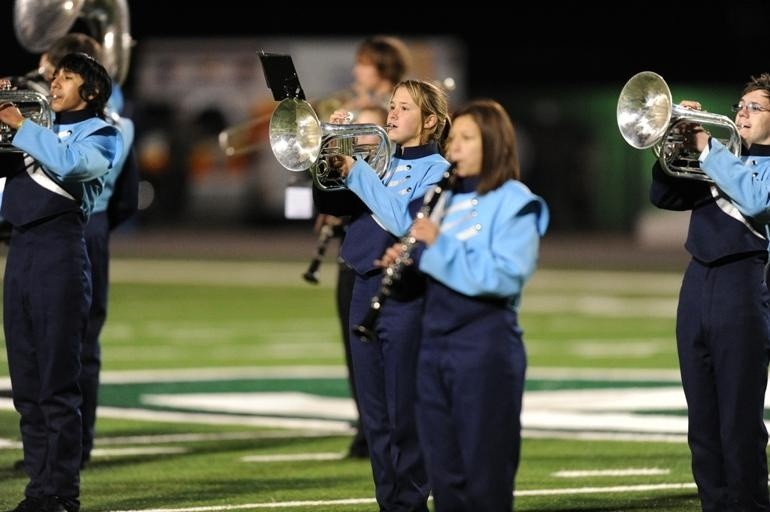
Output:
[16,499,79,512]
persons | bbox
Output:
[314,33,413,460]
[329,81,450,512]
[372,100,551,510]
[650,72,769,512]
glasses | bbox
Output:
[730,102,769,115]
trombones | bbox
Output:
[218,89,352,158]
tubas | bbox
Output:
[616,71,741,183]
[269,97,394,192]
[13,0,132,86]
[0,84,58,153]
